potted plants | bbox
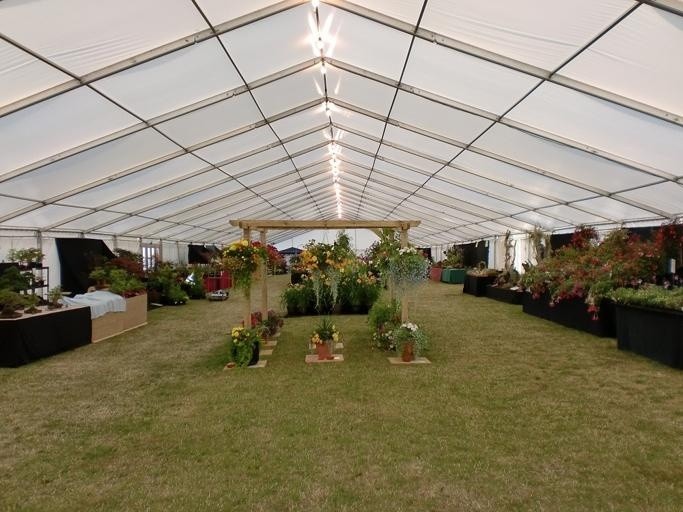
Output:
[0,266,63,319]
[7,246,45,269]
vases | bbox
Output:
[401,343,414,362]
[232,341,259,366]
[222,257,245,274]
[315,342,333,360]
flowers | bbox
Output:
[225,325,265,370]
[277,230,383,312]
[394,321,428,357]
[307,317,345,351]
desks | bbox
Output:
[441,268,466,283]
[91,293,148,343]
[204,277,218,291]
[218,271,231,289]
[429,267,442,281]
[487,286,530,304]
[463,274,497,297]
[0,305,92,368]
[606,302,683,372]
